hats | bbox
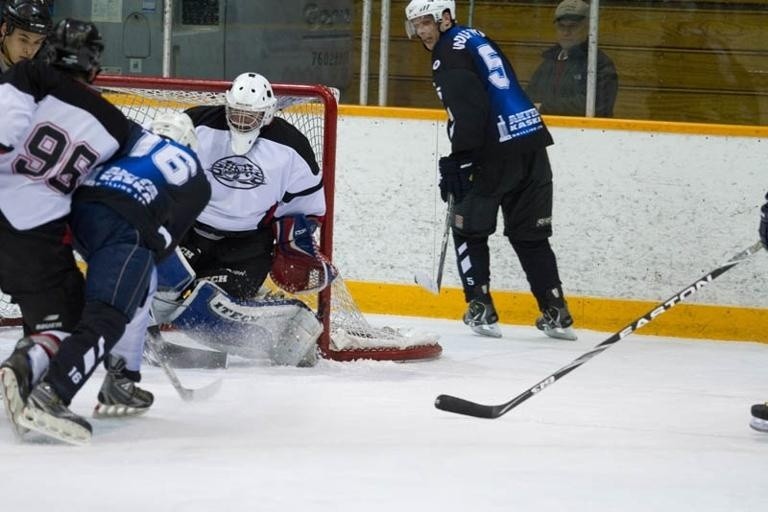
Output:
[552,0,591,23]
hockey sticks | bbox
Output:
[408,192,454,296]
[145,302,223,404]
[435,240,764,420]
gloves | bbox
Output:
[438,153,477,203]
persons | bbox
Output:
[15,111,212,445]
[0,0,51,75]
[748,189,768,431]
[0,17,133,437]
[404,0,578,342]
[141,72,327,369]
[525,0,619,119]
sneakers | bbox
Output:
[27,381,93,437]
[750,404,768,420]
[535,283,574,331]
[0,337,49,402]
[463,283,498,327]
[98,354,154,407]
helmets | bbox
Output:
[404,1,456,40]
[0,0,51,36]
[39,18,105,72]
[151,113,199,153]
[227,73,276,155]
